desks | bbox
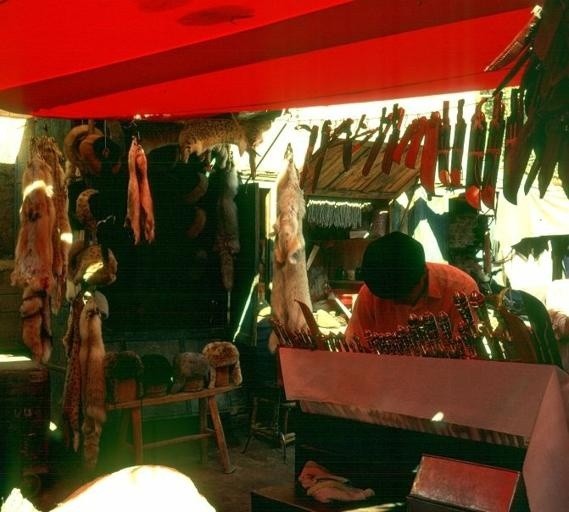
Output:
[105,383,241,472]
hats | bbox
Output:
[142,352,180,385]
[169,351,209,394]
[203,341,243,390]
[360,231,425,299]
[109,351,145,398]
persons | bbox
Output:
[336,232,481,358]
